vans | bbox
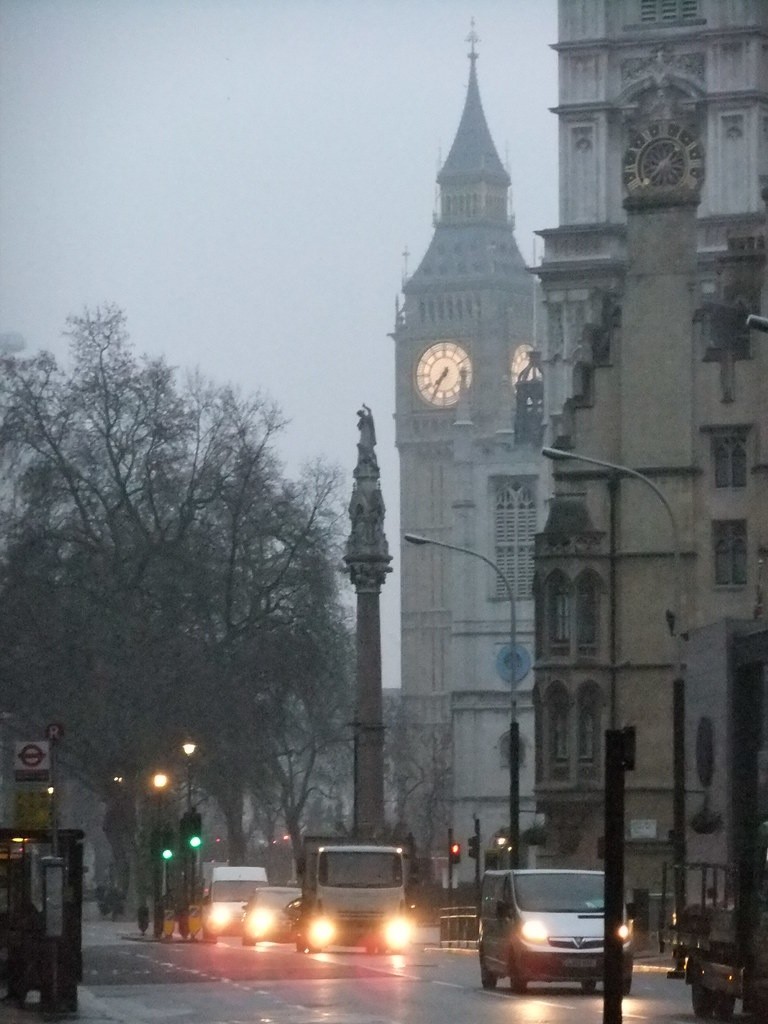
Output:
[477,866,634,998]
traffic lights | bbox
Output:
[187,815,202,849]
[162,830,174,862]
[450,845,463,865]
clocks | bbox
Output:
[510,344,543,405]
[416,340,474,406]
[639,136,689,187]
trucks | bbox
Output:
[299,834,406,957]
[677,614,768,1024]
[204,867,270,939]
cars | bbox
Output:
[240,887,303,946]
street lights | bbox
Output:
[152,772,171,895]
[540,445,689,957]
[403,532,522,873]
[182,740,199,907]
